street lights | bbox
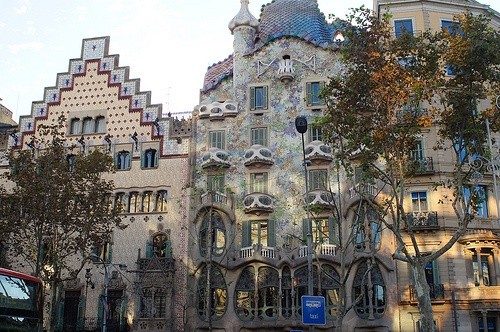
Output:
[295,116,314,331]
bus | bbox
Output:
[0,269,44,332]
